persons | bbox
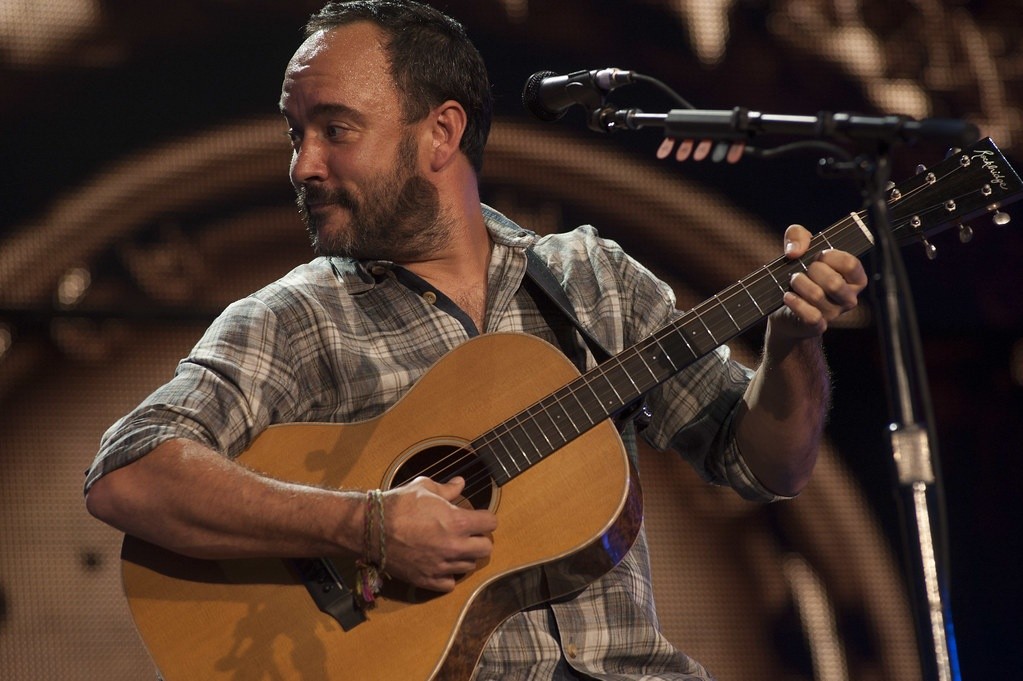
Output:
[86,0,869,680]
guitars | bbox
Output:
[120,136,1023,681]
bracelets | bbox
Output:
[355,489,385,598]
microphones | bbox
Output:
[522,68,634,124]
